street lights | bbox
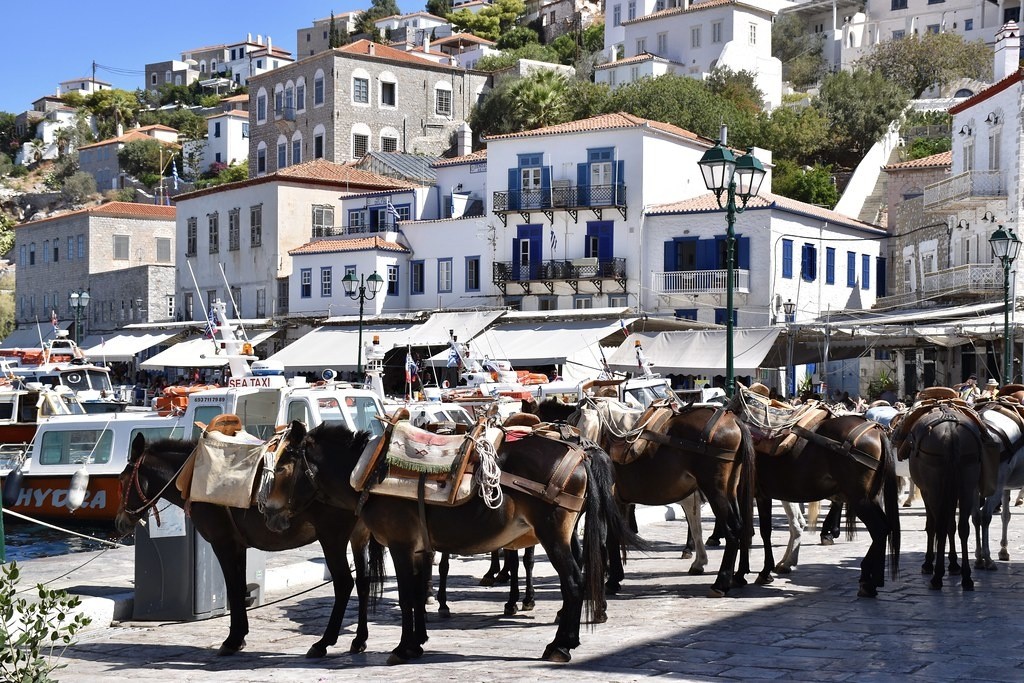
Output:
[988,225,1023,386]
[698,139,767,407]
[341,270,384,383]
[783,299,797,400]
[67,289,92,347]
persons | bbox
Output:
[952,374,999,404]
[769,386,854,408]
[879,383,900,406]
[549,368,558,383]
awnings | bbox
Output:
[139,328,281,373]
[78,328,187,364]
[250,314,642,382]
[605,327,782,378]
[0,320,74,348]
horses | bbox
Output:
[116,381,1024,664]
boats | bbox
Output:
[0,259,727,522]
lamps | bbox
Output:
[958,125,971,137]
[981,211,997,222]
[984,112,998,125]
[956,218,969,229]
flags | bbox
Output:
[387,202,401,219]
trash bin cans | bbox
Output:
[133,497,265,623]
